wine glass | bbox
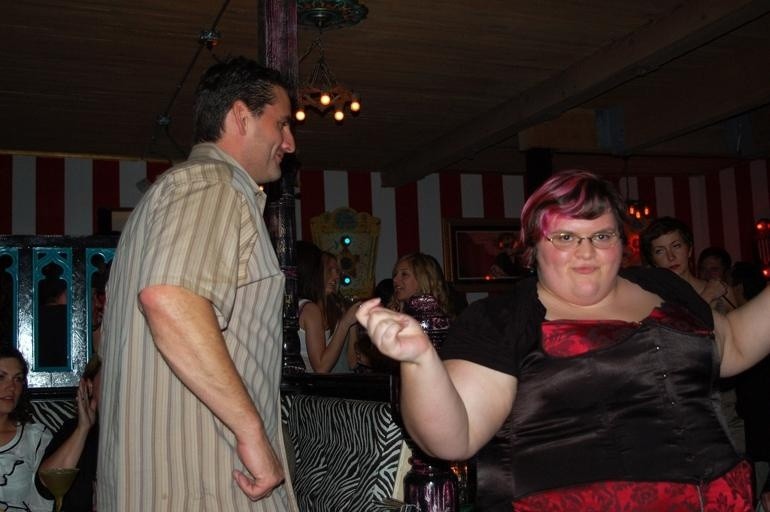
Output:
[40,469,78,512]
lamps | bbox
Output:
[292,0,368,124]
[627,198,656,228]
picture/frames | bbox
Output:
[451,222,523,283]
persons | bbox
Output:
[725,259,770,511]
[33,354,102,512]
[638,216,749,464]
[323,251,360,374]
[355,168,770,511]
[0,343,58,512]
[292,240,361,373]
[385,251,462,355]
[698,245,732,282]
[90,54,296,510]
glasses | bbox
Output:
[539,230,620,249]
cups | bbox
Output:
[343,296,359,306]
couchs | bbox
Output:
[27,371,413,512]
